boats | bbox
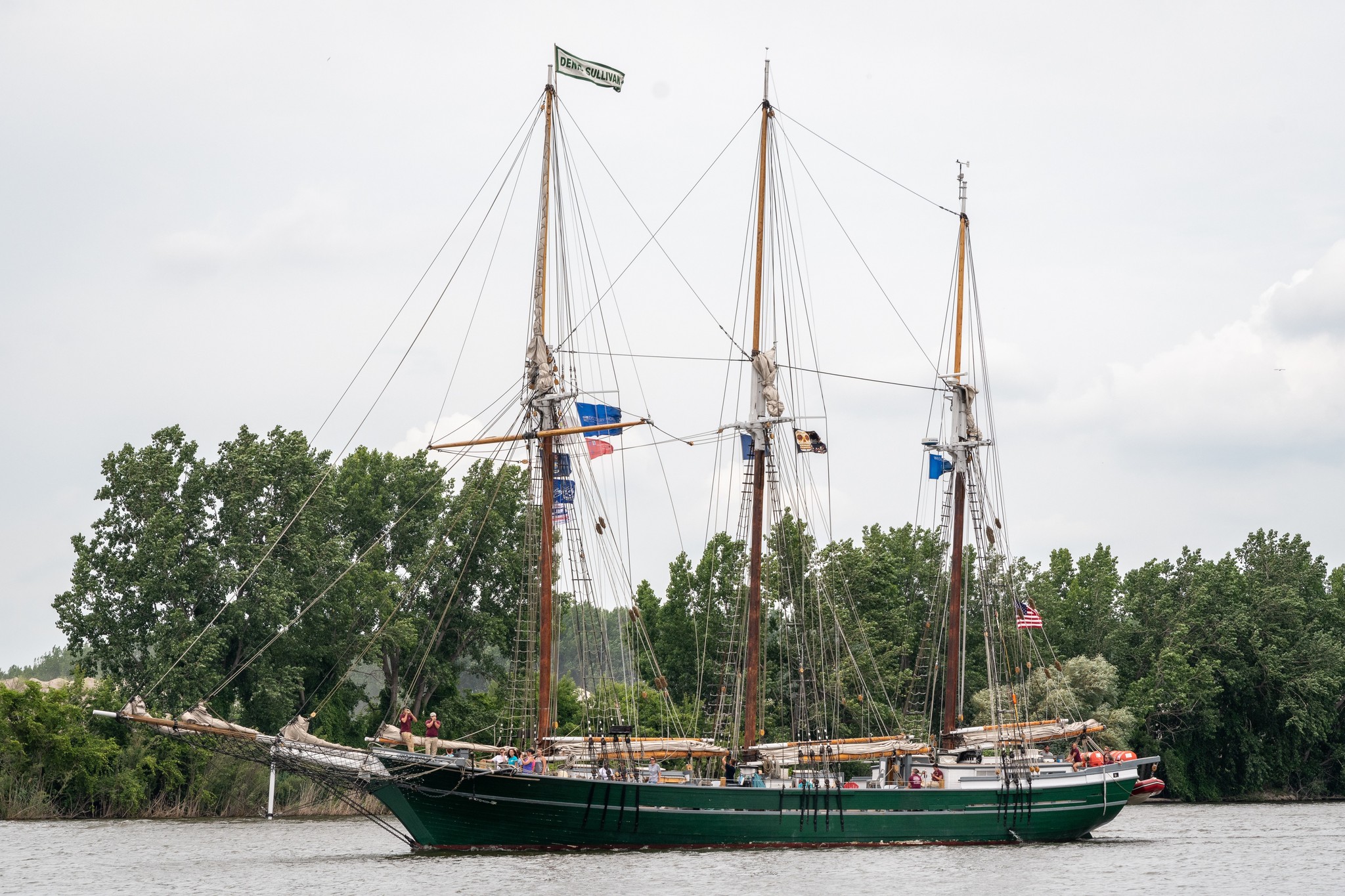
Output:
[87,45,1164,858]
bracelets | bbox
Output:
[724,756,726,758]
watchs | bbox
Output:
[434,721,436,722]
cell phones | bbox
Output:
[930,773,933,775]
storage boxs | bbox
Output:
[1043,758,1055,763]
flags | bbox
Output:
[740,433,773,460]
[929,453,954,479]
[539,403,623,527]
[1014,599,1043,630]
[553,43,625,92]
[792,428,828,454]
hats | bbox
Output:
[430,712,436,717]
[403,706,409,710]
[1044,745,1049,750]
[525,749,533,753]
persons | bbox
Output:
[597,756,662,785]
[425,713,441,756]
[444,745,547,777]
[721,755,737,787]
[400,706,418,753]
[901,763,944,790]
[1036,740,1123,774]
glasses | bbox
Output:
[500,751,504,753]
[527,752,530,753]
[1104,757,1109,759]
[431,716,435,717]
[1104,750,1109,751]
[651,759,655,761]
